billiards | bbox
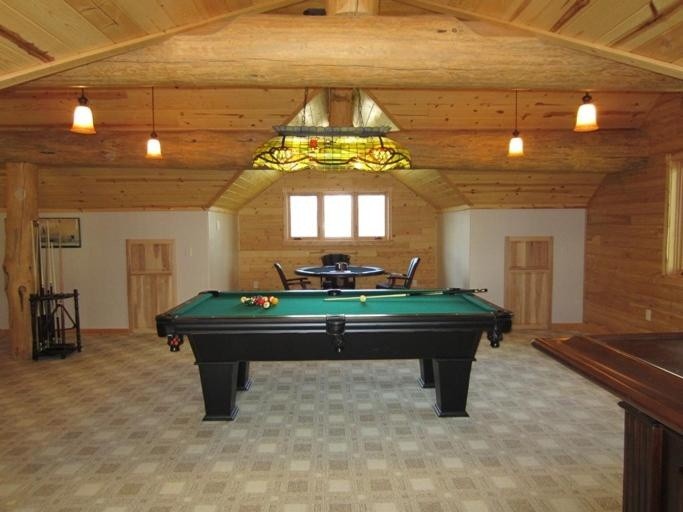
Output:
[360,295,366,301]
[241,296,278,309]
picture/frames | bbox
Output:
[38,217,81,248]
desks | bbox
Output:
[154,290,516,417]
[294,265,385,290]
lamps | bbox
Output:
[69,87,97,135]
[144,87,162,160]
[508,91,525,159]
[574,92,599,134]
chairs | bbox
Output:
[376,256,421,290]
[274,263,316,290]
[319,253,356,288]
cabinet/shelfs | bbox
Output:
[29,288,82,359]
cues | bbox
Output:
[326,289,487,300]
[31,219,66,351]
